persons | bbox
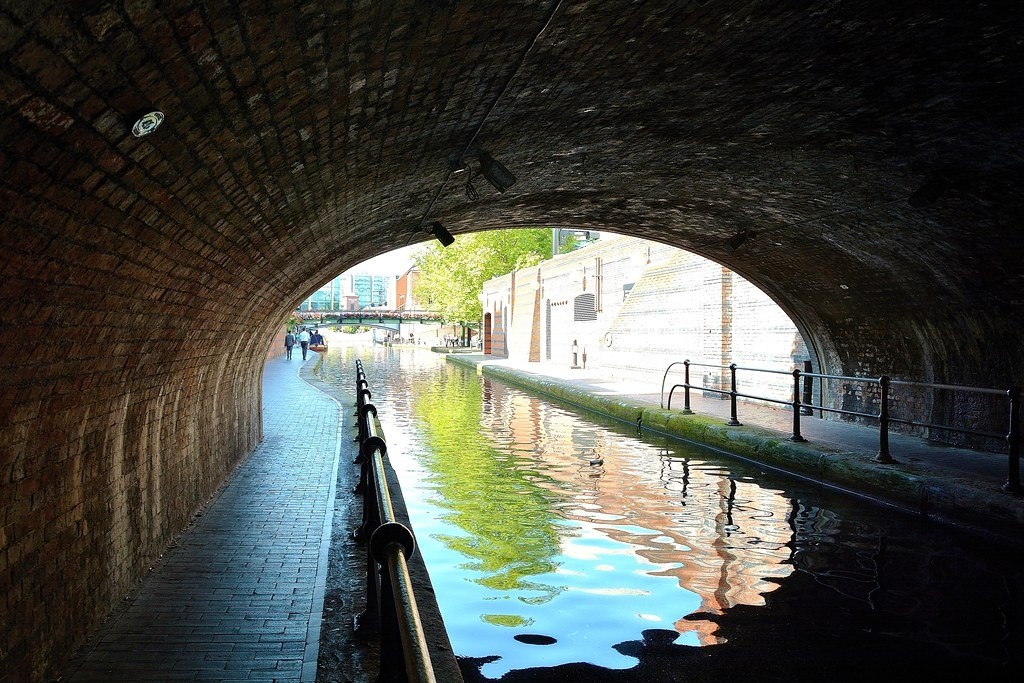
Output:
[300,328,310,360]
[284,331,294,360]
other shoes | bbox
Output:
[303,358,307,360]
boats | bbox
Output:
[309,335,329,351]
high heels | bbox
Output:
[290,358,292,360]
